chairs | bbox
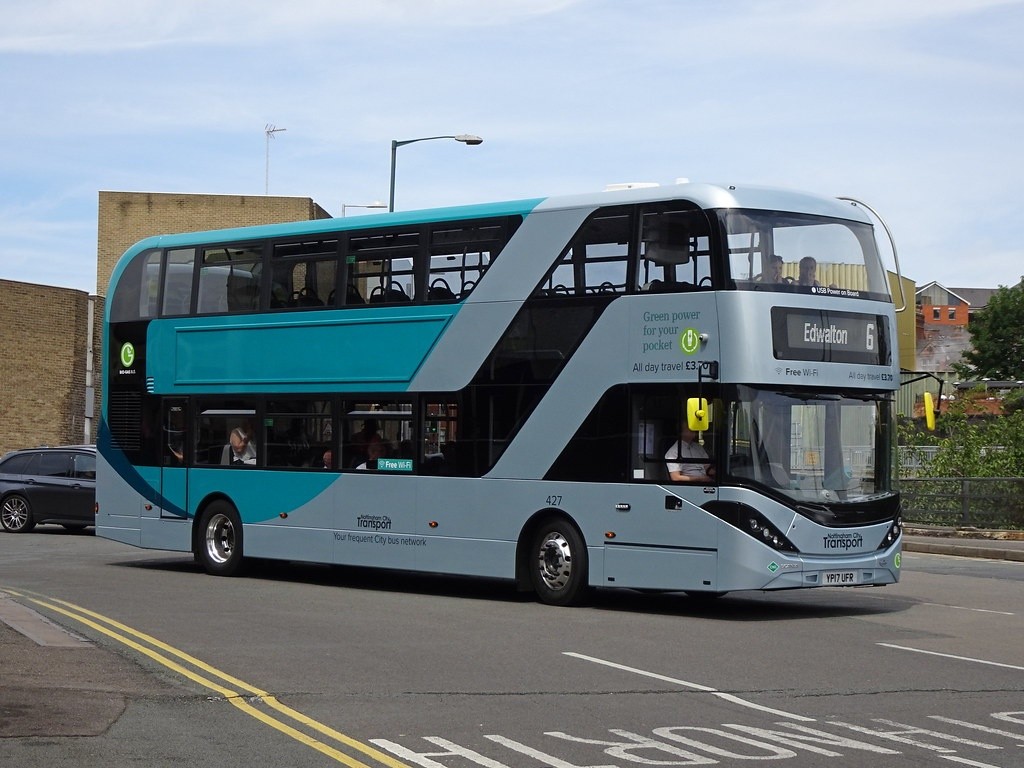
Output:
[268,279,619,307]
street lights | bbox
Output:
[390,134,483,214]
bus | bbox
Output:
[90,177,945,610]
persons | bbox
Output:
[664,414,719,483]
[791,257,838,290]
[751,254,791,292]
[229,416,257,466]
[351,418,415,473]
[278,416,310,462]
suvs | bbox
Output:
[0,444,97,534]
